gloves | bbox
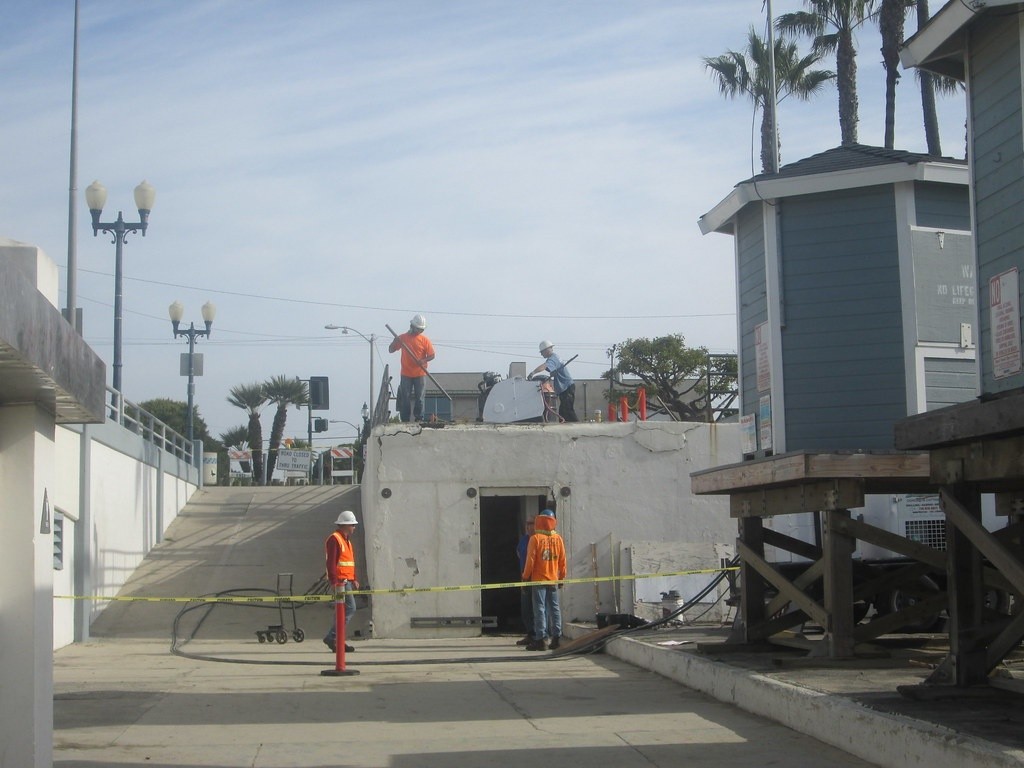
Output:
[558,579,563,589]
[528,373,533,381]
[522,580,528,590]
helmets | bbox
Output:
[540,509,555,518]
[334,511,358,525]
[410,314,425,329]
[538,341,555,352]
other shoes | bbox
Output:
[333,643,354,652]
[549,637,560,648]
[323,636,337,652]
[517,636,535,645]
[526,640,546,651]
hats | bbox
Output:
[525,515,535,523]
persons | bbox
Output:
[516,515,536,644]
[522,510,566,651]
[527,340,580,422]
[323,511,360,654]
[389,312,437,423]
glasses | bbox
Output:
[349,524,355,527]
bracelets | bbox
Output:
[529,373,533,376]
